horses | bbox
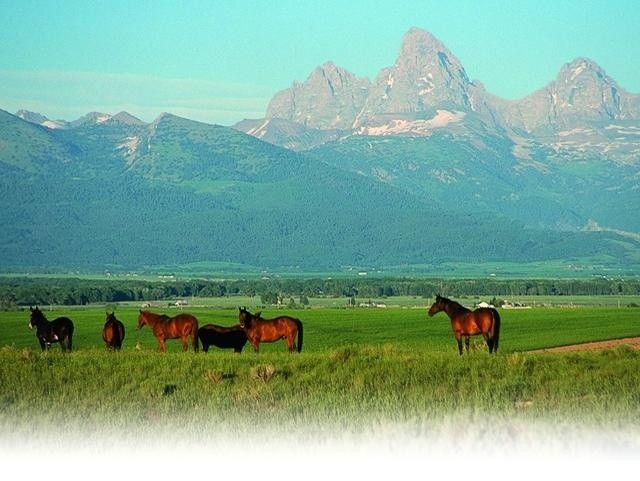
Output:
[238,306,303,354]
[135,309,199,352]
[28,305,74,352]
[197,312,261,354]
[102,311,125,352]
[428,292,501,356]
